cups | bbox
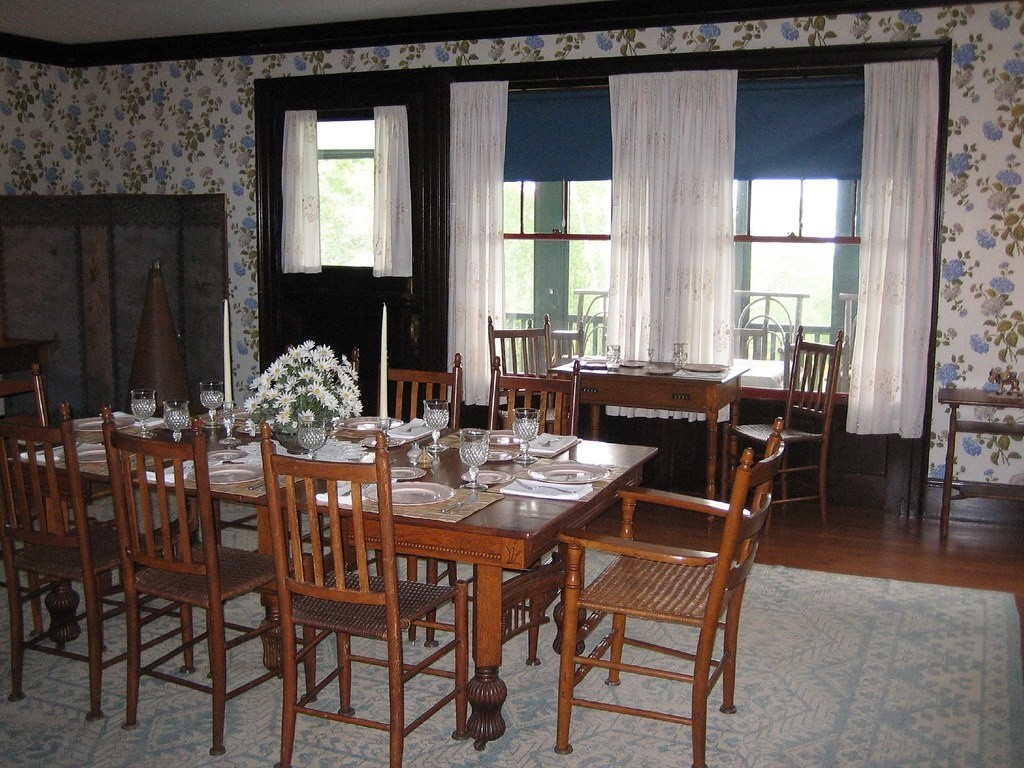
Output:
[606,345,621,370]
[672,342,689,368]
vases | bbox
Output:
[273,419,327,455]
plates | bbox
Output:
[527,464,610,483]
[336,416,406,448]
[365,482,456,506]
[187,450,264,484]
[59,416,135,463]
[220,408,262,434]
[390,466,427,480]
[584,361,726,374]
[461,434,521,484]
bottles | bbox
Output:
[131,389,158,438]
[407,442,434,468]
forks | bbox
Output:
[534,437,562,447]
[515,479,575,494]
[401,424,425,433]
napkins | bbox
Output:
[142,460,192,482]
[19,443,82,466]
[387,417,434,440]
[673,370,729,382]
[315,479,398,510]
[502,478,594,503]
[579,355,610,361]
[102,410,164,429]
[528,431,578,455]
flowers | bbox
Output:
[244,342,363,423]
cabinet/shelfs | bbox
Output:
[549,356,749,533]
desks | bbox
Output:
[937,388,1024,541]
[42,412,663,751]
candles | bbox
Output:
[376,305,391,420]
[223,298,234,401]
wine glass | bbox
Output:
[423,398,449,451]
[162,399,189,442]
[512,408,540,464]
[199,380,224,428]
[218,401,241,444]
[459,428,490,491]
[297,416,327,460]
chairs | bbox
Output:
[0,291,862,768]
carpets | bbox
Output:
[0,479,1024,768]
